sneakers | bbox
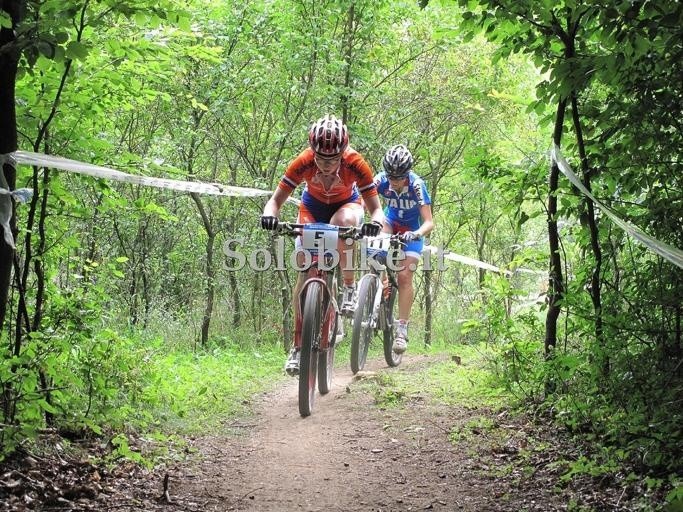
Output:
[392,334,409,354]
[286,346,301,375]
[339,282,358,315]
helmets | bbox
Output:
[308,115,349,160]
[383,144,414,180]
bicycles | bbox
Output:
[343,231,421,376]
[256,215,374,418]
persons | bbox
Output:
[258,112,385,372]
[359,143,434,354]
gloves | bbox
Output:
[262,216,278,231]
[361,221,383,237]
[402,231,422,241]
